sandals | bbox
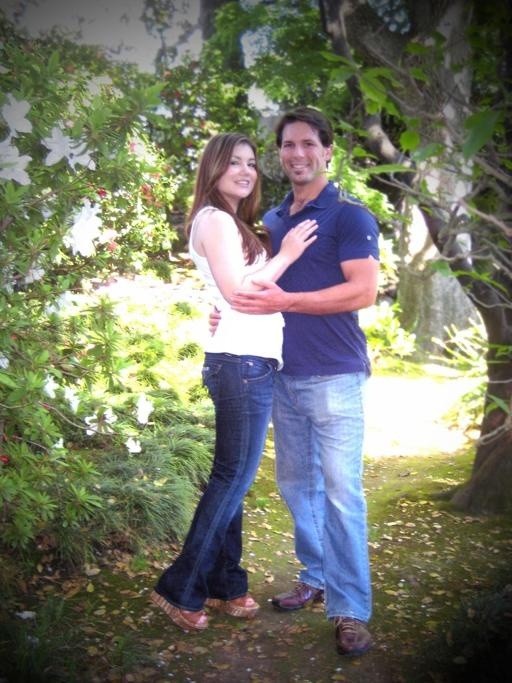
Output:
[149,590,260,631]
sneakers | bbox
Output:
[334,616,372,657]
[271,582,323,611]
